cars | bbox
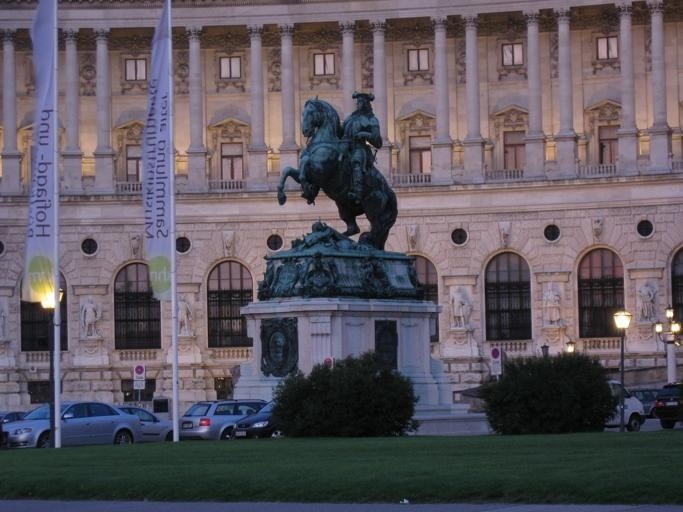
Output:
[626,386,662,420]
[0,397,288,451]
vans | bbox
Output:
[600,377,646,433]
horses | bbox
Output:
[276,94,400,251]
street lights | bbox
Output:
[539,341,549,357]
[39,282,64,450]
[564,337,577,357]
[654,303,682,383]
[611,303,634,434]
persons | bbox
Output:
[342,90,383,200]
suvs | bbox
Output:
[650,381,682,430]
